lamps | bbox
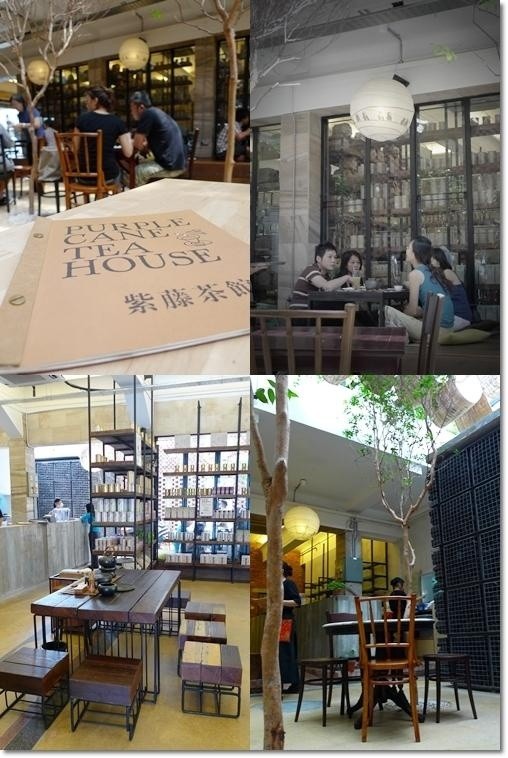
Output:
[283,478,320,540]
[27,57,49,86]
[118,12,150,71]
[349,26,416,143]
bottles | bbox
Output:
[326,114,501,307]
[88,568,96,593]
[2,513,8,526]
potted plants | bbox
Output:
[326,581,346,595]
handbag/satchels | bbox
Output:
[37,146,61,182]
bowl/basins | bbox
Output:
[98,582,118,595]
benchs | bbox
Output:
[190,158,249,182]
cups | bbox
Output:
[93,453,250,569]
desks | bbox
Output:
[251,325,409,375]
[307,285,408,327]
[322,619,436,729]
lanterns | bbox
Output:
[28,60,49,84]
[284,506,321,543]
[0,82,17,100]
[351,79,416,142]
[119,37,150,72]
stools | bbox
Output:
[294,656,351,727]
[421,653,478,723]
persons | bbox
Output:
[81,504,102,569]
[388,576,407,617]
[333,250,378,328]
[428,248,471,333]
[281,561,301,693]
[289,243,351,325]
[1,89,248,198]
[51,498,71,522]
[381,235,455,346]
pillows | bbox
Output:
[436,328,493,345]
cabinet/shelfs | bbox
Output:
[159,444,250,583]
[89,429,159,570]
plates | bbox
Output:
[116,582,136,593]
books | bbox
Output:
[0,210,248,374]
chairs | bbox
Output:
[251,302,358,374]
[354,593,420,742]
[326,611,376,708]
[405,292,445,376]
[0,128,200,216]
